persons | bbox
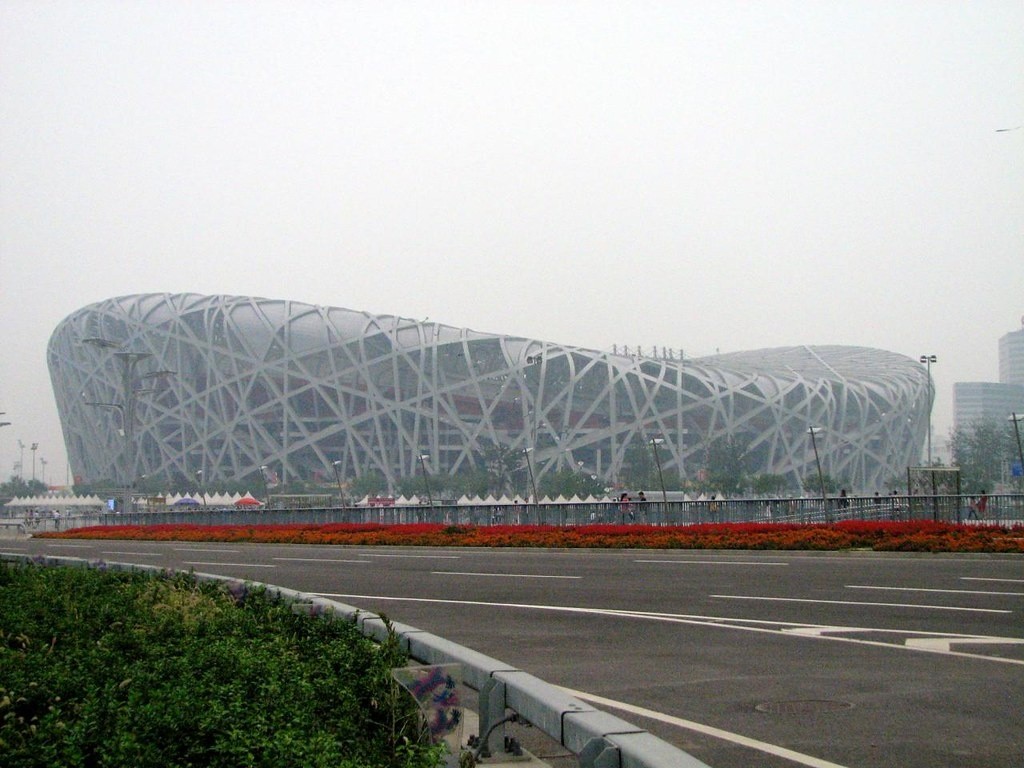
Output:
[888,490,900,520]
[837,489,848,520]
[513,497,530,504]
[709,495,717,512]
[619,492,647,511]
[967,489,988,520]
[23,508,103,530]
[873,492,881,508]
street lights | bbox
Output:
[418,453,433,507]
[650,436,667,520]
[195,469,206,509]
[1008,412,1024,473]
[920,355,937,467]
[332,459,344,509]
[259,465,271,509]
[806,425,829,523]
[523,446,543,524]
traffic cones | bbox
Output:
[30,443,38,481]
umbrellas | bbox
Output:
[172,498,199,508]
[234,497,261,506]
